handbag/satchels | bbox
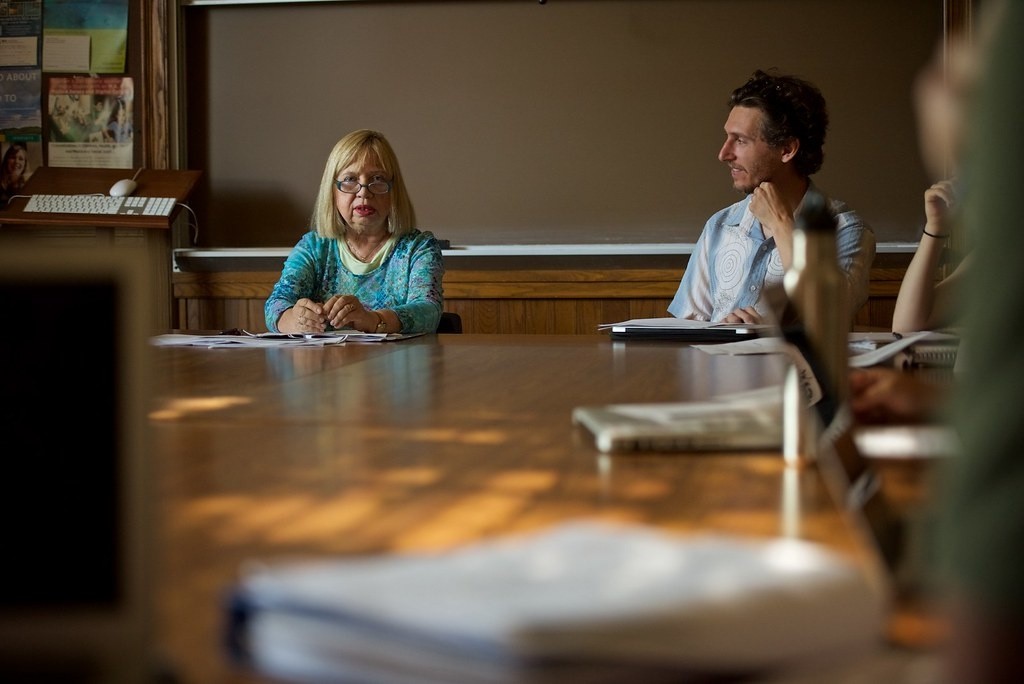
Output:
[214,516,876,684]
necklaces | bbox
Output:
[346,239,385,261]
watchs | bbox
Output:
[364,311,386,334]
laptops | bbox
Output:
[609,325,760,342]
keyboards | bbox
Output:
[24,195,177,217]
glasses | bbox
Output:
[333,176,394,195]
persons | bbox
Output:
[906,0,1024,684]
[264,130,445,333]
[0,143,32,204]
[893,175,975,334]
[666,69,876,328]
[51,94,132,142]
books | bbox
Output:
[224,522,875,684]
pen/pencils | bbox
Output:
[765,279,902,583]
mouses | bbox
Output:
[109,179,138,197]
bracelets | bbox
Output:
[923,229,950,238]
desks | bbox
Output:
[145,333,966,684]
